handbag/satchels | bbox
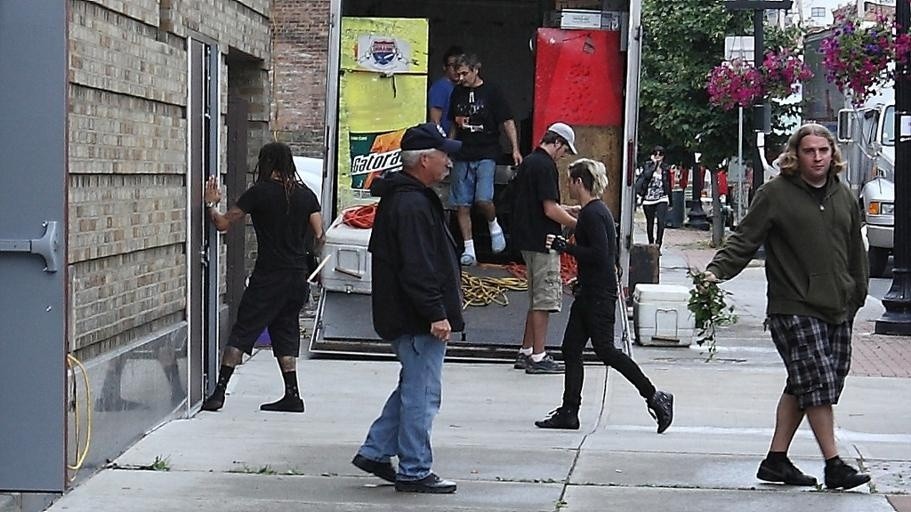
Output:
[635,173,649,197]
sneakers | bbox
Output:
[394,472,457,494]
[261,398,304,413]
[460,252,478,266]
[535,407,580,429]
[352,454,397,482]
[490,230,506,255]
[525,353,567,374]
[757,457,816,487]
[201,393,224,411]
[513,352,531,369]
[646,392,674,433]
[824,463,871,489]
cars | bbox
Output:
[666,183,737,232]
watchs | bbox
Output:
[205,200,215,208]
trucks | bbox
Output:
[305,0,647,367]
[825,96,894,277]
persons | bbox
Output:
[497,122,580,374]
[533,157,674,435]
[696,123,869,490]
[450,51,522,266]
[634,146,672,253]
[350,122,462,494]
[197,142,322,412]
[428,47,464,210]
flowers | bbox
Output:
[758,52,814,98]
[735,64,761,112]
[820,7,911,109]
[705,62,738,113]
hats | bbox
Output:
[548,121,579,156]
[400,121,462,155]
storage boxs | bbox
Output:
[319,205,375,291]
[634,282,695,349]
